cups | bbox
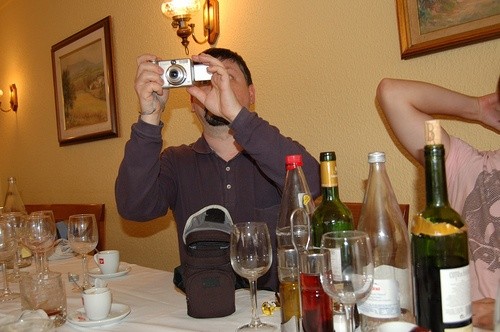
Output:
[80,287,112,321]
[94,250,120,274]
[17,271,67,327]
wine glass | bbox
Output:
[0,210,99,303]
[229,221,277,332]
[318,230,374,332]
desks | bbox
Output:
[0,254,362,332]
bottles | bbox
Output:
[409,144,473,328]
[276,155,318,332]
[312,151,356,315]
[274,243,338,332]
[3,176,27,215]
[350,151,419,332]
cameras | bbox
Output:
[152,58,214,89]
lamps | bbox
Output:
[0,83,18,112]
[160,0,219,55]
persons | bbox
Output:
[376,77,499,332]
[116,49,323,293]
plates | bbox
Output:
[67,303,131,326]
[89,261,131,279]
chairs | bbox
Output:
[25,203,106,257]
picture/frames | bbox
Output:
[396,0,500,60]
[50,15,120,147]
